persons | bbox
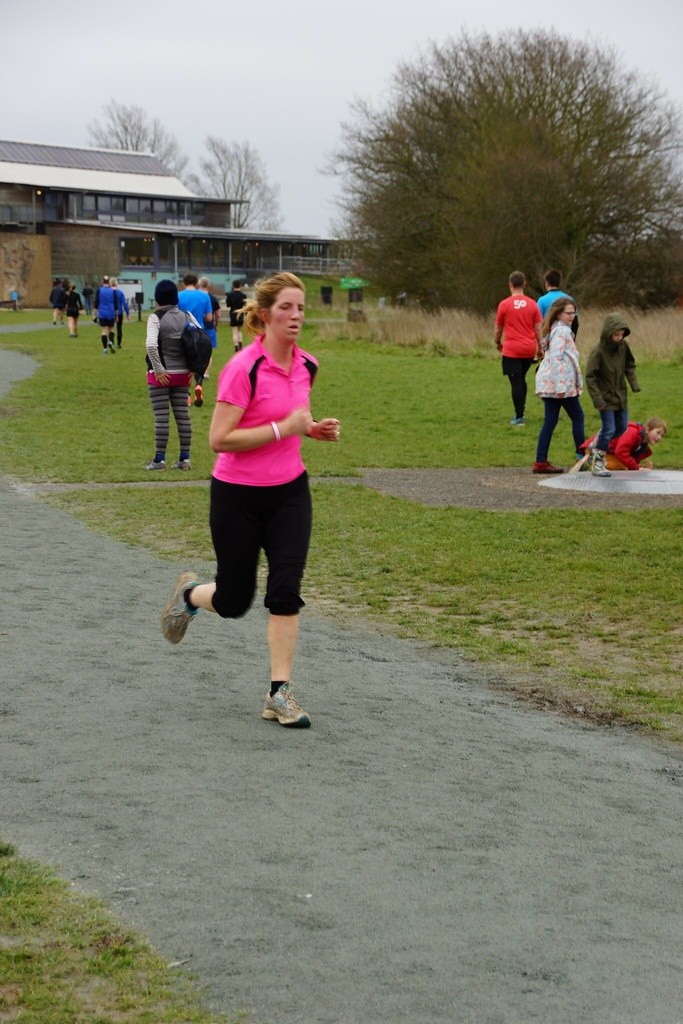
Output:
[225,280,247,353]
[534,298,590,472]
[163,271,341,728]
[537,268,579,342]
[579,417,666,470]
[49,279,85,338]
[110,277,130,348]
[197,277,219,378]
[92,276,119,353]
[145,280,201,471]
[585,312,641,477]
[493,271,545,426]
[82,282,94,315]
[178,273,213,407]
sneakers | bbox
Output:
[171,459,192,470]
[141,459,167,471]
[161,572,202,644]
[576,460,593,472]
[532,460,564,473]
[262,681,311,727]
[511,416,525,426]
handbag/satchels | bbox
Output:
[179,313,212,378]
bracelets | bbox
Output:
[495,339,500,344]
[270,421,281,441]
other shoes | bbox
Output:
[107,341,115,353]
[193,385,204,408]
[188,398,192,406]
[104,349,108,354]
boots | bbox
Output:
[592,451,611,477]
